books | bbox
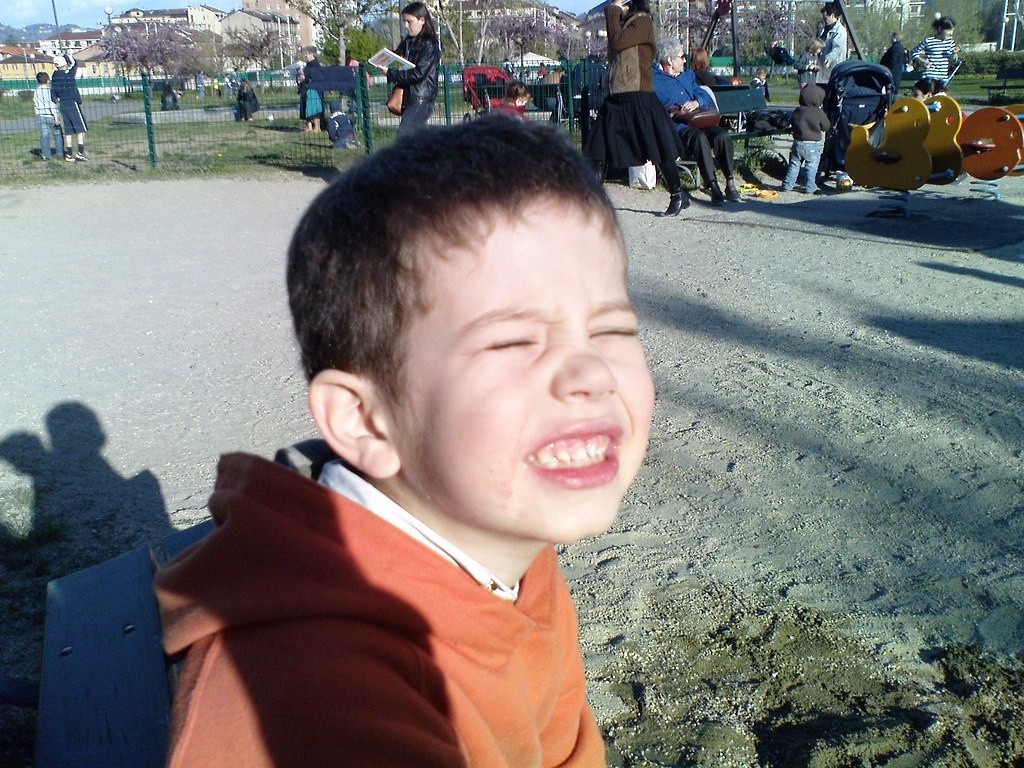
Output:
[367,47,415,74]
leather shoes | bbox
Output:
[658,187,691,216]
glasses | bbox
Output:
[677,53,687,60]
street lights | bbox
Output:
[584,29,608,56]
[105,6,116,65]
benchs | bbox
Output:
[675,89,800,189]
[980,70,1024,104]
[36,518,221,768]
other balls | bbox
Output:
[836,173,853,190]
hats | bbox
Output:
[53,55,69,68]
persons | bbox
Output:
[296,45,323,133]
[690,47,716,86]
[345,49,373,91]
[879,32,904,105]
[494,80,530,122]
[234,78,261,122]
[161,84,180,111]
[33,53,89,162]
[769,39,795,66]
[154,114,654,768]
[794,38,825,90]
[750,67,771,102]
[296,68,305,86]
[910,16,962,85]
[904,48,910,64]
[584,0,691,217]
[195,68,239,100]
[782,84,830,193]
[502,53,605,85]
[650,37,742,206]
[326,100,365,150]
[378,2,440,149]
[913,77,967,126]
[814,6,847,112]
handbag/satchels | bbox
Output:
[669,105,721,129]
[386,84,406,116]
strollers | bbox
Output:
[455,65,526,124]
[821,60,897,175]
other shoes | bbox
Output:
[76,151,90,161]
[724,184,742,202]
[709,190,723,206]
[64,154,75,161]
